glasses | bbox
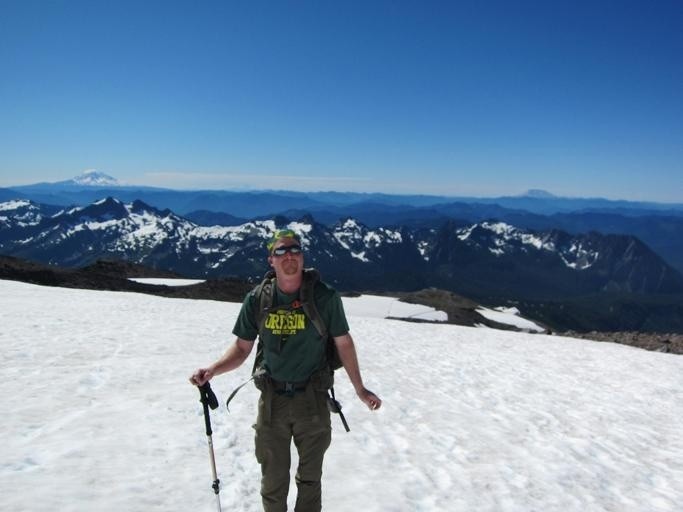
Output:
[271,244,301,256]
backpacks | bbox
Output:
[252,267,343,394]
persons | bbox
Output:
[189,227,381,511]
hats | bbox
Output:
[267,229,299,250]
